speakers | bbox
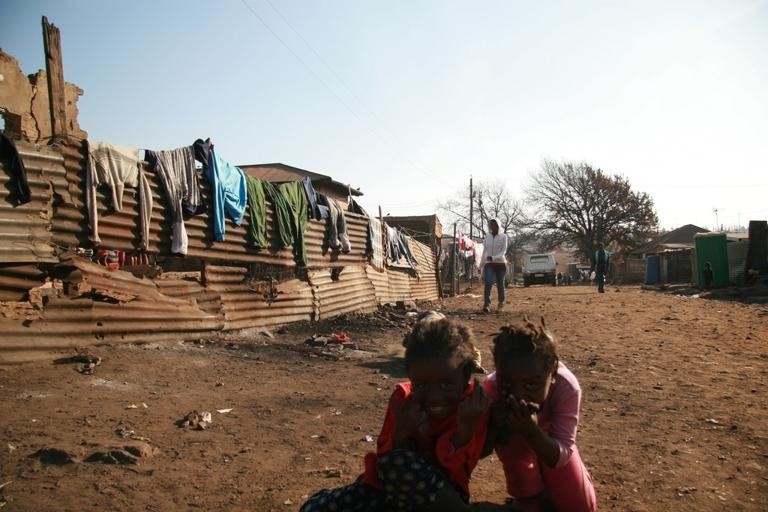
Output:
[483,302,492,313]
[495,300,505,314]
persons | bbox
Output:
[589,242,610,293]
[477,217,508,314]
[477,316,596,512]
[293,308,486,511]
